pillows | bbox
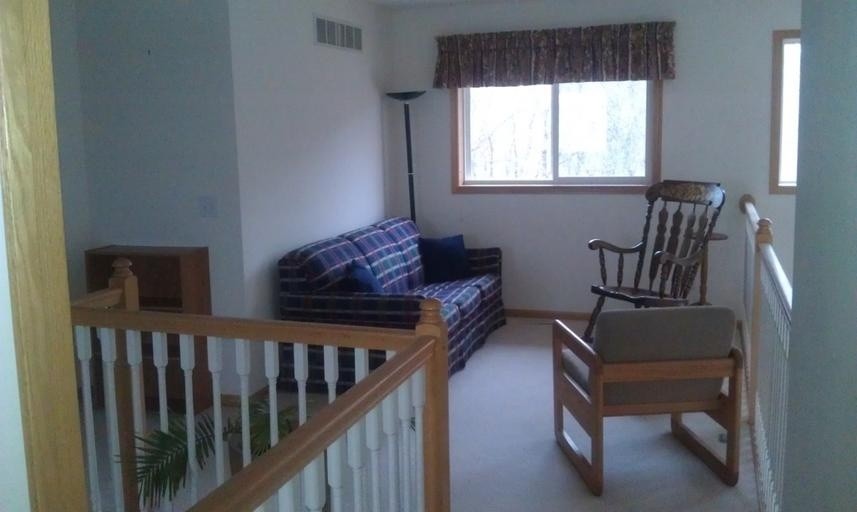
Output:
[341,258,386,295]
[417,233,472,288]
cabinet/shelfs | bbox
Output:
[83,244,213,414]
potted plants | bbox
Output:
[110,394,314,512]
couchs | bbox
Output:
[275,215,507,392]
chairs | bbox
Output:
[580,178,731,345]
[554,302,747,498]
